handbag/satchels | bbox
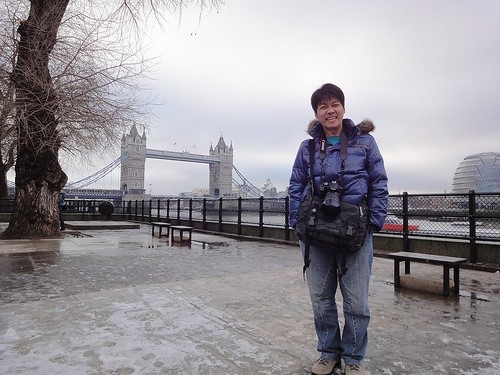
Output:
[296,187,368,251]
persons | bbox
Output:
[288,82,389,375]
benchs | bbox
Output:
[387,251,469,297]
[168,226,194,244]
[151,222,171,238]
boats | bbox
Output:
[381,215,419,231]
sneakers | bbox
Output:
[312,356,340,375]
[344,362,362,375]
